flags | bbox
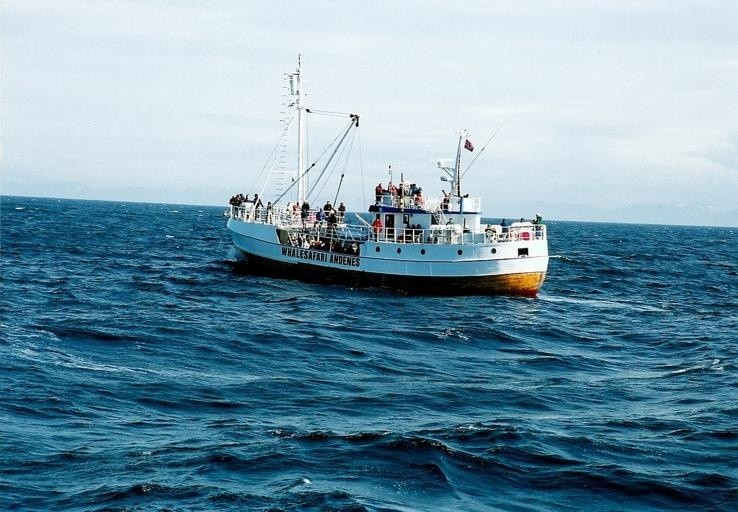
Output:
[464,138,475,153]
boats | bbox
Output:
[226,53,552,300]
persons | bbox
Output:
[531,214,543,238]
[446,217,454,244]
[229,179,423,253]
[501,219,507,232]
